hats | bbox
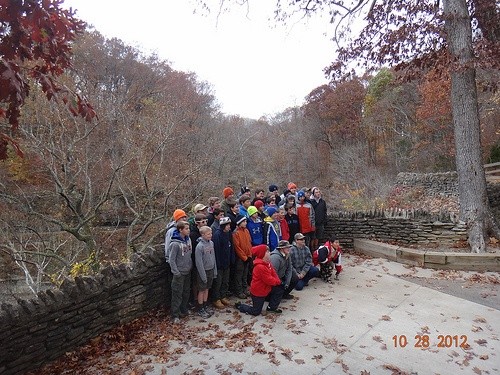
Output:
[298,191,305,200]
[268,185,278,192]
[236,215,247,226]
[174,209,187,222]
[288,182,296,190]
[219,217,231,224]
[248,206,258,216]
[276,240,293,249]
[195,203,208,213]
[254,200,264,208]
[294,233,306,239]
[301,187,311,192]
[223,187,234,198]
[250,245,267,259]
[267,207,278,217]
[238,186,250,196]
[283,189,291,197]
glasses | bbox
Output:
[196,219,208,224]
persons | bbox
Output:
[290,233,320,291]
[234,245,284,316]
[270,240,299,300]
[165,181,327,319]
[313,238,342,285]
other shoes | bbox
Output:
[234,301,241,309]
[234,291,250,299]
[213,298,230,309]
[193,305,214,319]
[266,305,282,313]
[172,317,180,324]
[185,309,194,317]
[282,293,294,299]
